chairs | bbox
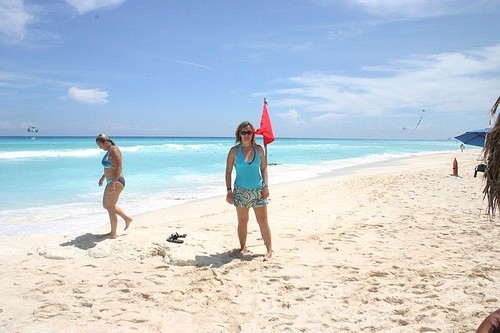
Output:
[474,163,487,177]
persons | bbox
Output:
[225,120,274,262]
[476,97,500,333]
[459,144,465,153]
[95,134,134,240]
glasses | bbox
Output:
[239,130,254,135]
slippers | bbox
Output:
[168,236,184,243]
[169,232,186,238]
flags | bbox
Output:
[250,96,275,148]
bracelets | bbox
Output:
[263,184,269,188]
[227,188,232,191]
